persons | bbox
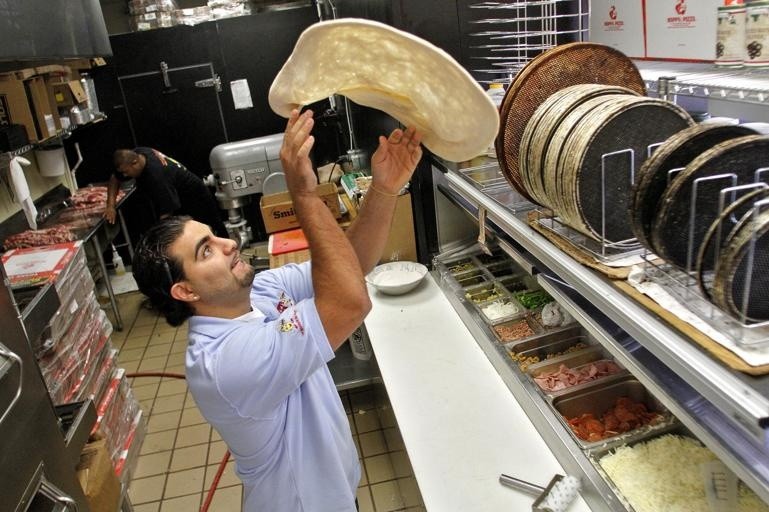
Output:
[132,102,422,511]
[102,146,228,238]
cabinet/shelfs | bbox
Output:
[364,62,768,511]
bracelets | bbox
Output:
[370,183,400,198]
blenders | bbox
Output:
[201,133,297,270]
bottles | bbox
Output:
[111,252,128,278]
[484,81,512,161]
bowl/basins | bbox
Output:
[364,260,428,294]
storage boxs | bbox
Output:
[259,180,341,233]
[73,439,122,512]
[0,61,88,150]
